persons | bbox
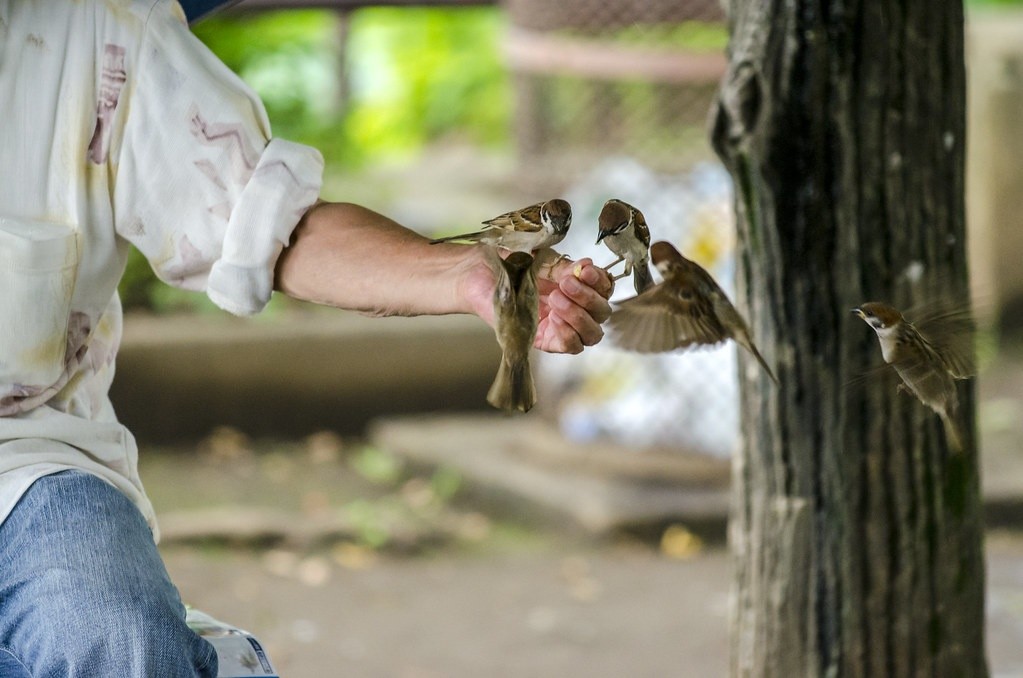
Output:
[0,0,615,678]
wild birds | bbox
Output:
[490,250,538,413]
[593,197,656,295]
[604,241,780,388]
[429,198,573,258]
[850,298,996,453]
